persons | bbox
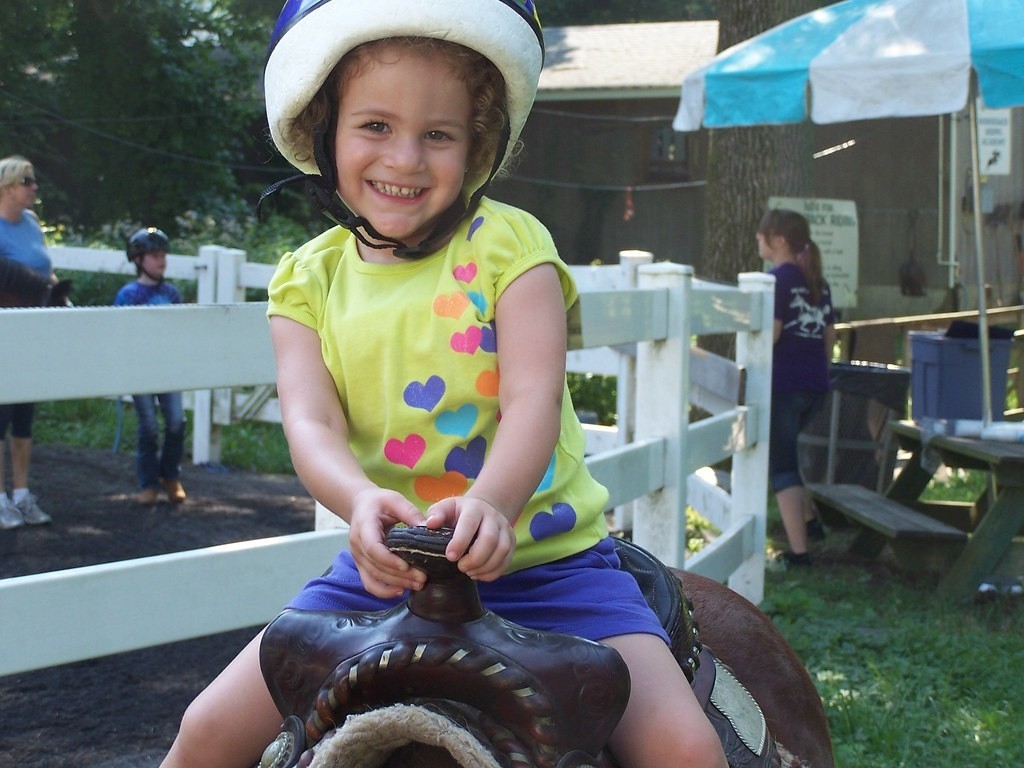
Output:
[115,227,187,504]
[155,0,731,768]
[757,209,836,566]
[0,156,74,528]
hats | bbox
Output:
[264,0,544,180]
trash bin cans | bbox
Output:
[907,320,1015,428]
[801,361,911,490]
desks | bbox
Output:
[840,420,1024,600]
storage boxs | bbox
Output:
[907,329,1014,422]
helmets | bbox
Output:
[126,227,171,259]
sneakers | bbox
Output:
[0,493,52,529]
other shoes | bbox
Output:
[775,549,813,565]
[140,488,157,502]
[806,518,825,539]
[159,478,185,502]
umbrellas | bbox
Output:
[672,0,1024,509]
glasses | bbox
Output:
[21,176,39,185]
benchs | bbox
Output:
[804,483,967,541]
